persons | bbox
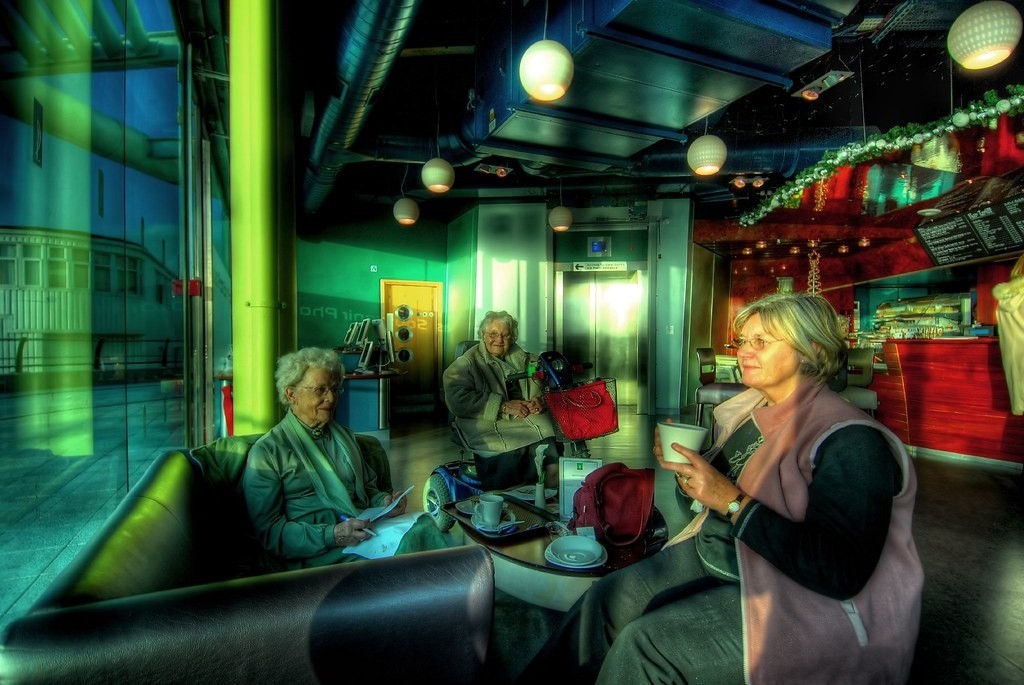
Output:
[443,311,564,495]
[992,253,1024,416]
[240,345,450,570]
[518,290,923,685]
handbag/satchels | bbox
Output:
[545,380,618,439]
[566,462,655,546]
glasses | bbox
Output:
[732,338,783,351]
[289,385,344,400]
[487,332,511,339]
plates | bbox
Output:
[471,513,516,533]
[544,540,607,568]
[551,536,603,566]
[455,499,508,514]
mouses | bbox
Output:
[353,371,363,375]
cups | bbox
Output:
[657,421,709,464]
[474,494,504,530]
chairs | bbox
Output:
[692,346,749,443]
[839,346,877,418]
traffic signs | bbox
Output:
[573,261,628,271]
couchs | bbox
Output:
[0,423,516,685]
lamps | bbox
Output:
[947,0,1024,69]
[687,112,728,176]
[393,163,420,228]
[420,84,457,195]
[789,49,855,101]
[547,176,574,233]
[475,163,514,177]
[728,170,770,188]
[518,0,577,105]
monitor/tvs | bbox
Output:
[344,319,395,374]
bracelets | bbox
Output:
[503,402,507,413]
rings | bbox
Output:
[360,538,364,542]
[676,472,685,480]
[682,475,690,486]
[536,408,539,411]
[652,446,658,457]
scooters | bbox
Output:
[423,339,620,533]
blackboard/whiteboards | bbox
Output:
[964,193,1024,255]
[911,214,987,266]
[915,177,988,226]
[964,165,1024,214]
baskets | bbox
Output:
[540,377,619,442]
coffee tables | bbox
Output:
[457,484,618,614]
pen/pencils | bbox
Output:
[340,514,377,537]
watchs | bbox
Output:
[725,492,746,519]
